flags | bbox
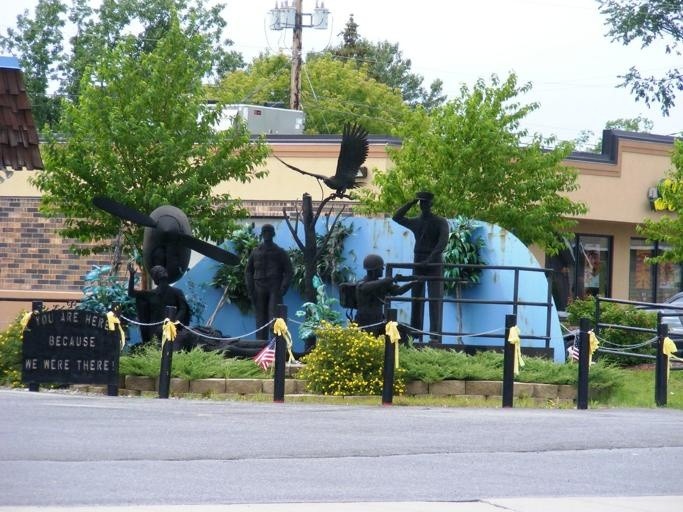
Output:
[573,333,580,359]
[253,335,276,372]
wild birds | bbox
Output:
[271,121,369,199]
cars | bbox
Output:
[563,303,682,363]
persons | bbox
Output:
[391,192,450,343]
[353,254,424,346]
[152,321,315,362]
[126,260,190,358]
[245,222,294,339]
[550,259,573,312]
